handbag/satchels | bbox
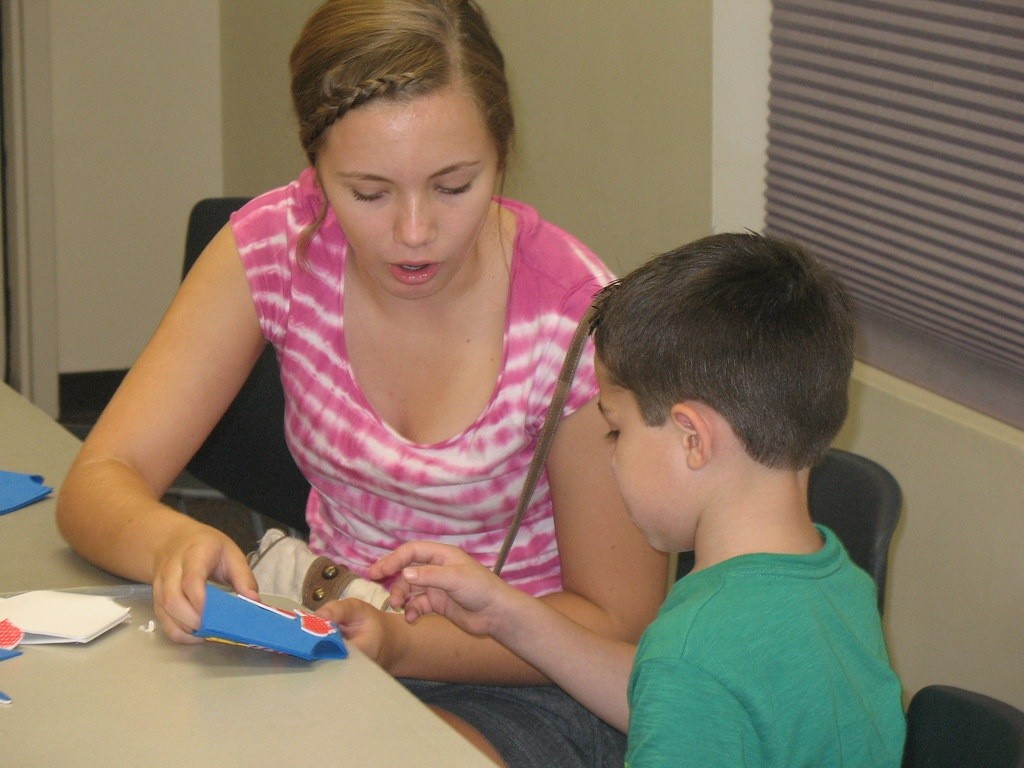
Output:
[246,527,406,644]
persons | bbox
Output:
[369,232,908,768]
[57,0,671,768]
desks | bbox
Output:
[0,377,505,768]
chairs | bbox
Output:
[46,193,315,534]
[678,448,1023,766]
